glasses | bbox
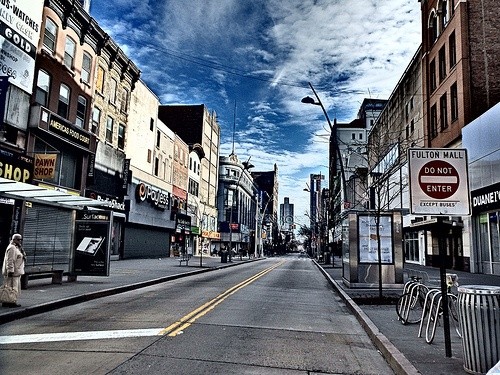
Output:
[16,239,23,241]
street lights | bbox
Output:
[301,82,348,210]
[229,156,255,261]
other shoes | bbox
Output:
[2,303,9,307]
[9,303,22,308]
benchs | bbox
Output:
[176,254,193,266]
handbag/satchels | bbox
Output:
[0,276,18,303]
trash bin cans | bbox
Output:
[457,285,500,375]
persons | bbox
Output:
[2,233,24,307]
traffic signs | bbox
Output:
[408,149,470,216]
